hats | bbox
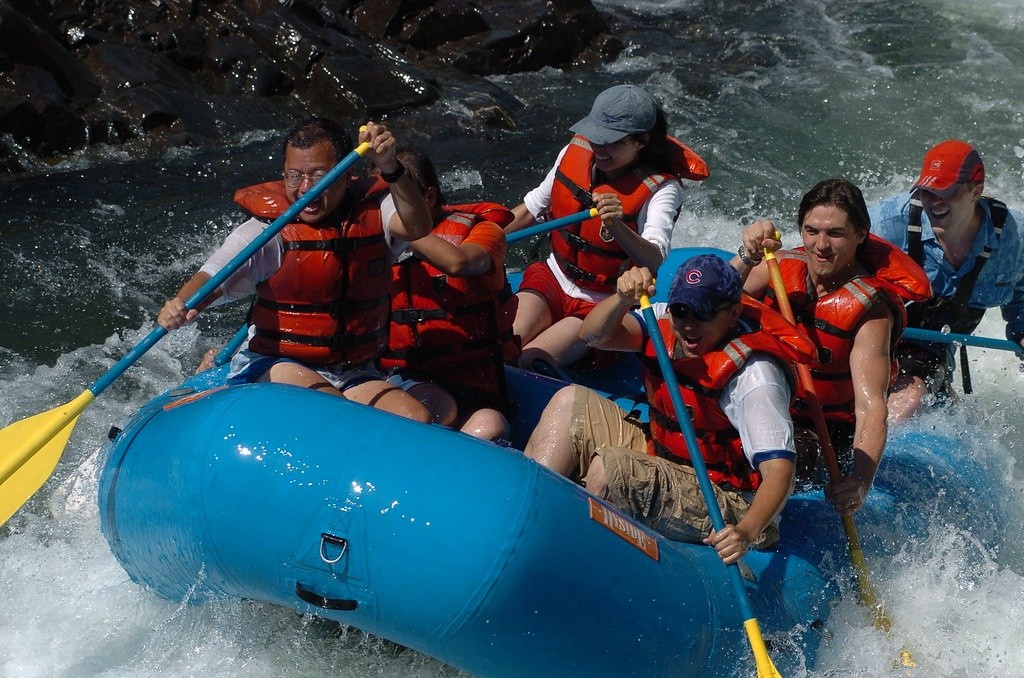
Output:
[665,253,741,320]
[568,84,657,145]
[909,139,984,199]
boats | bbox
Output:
[98,247,1006,678]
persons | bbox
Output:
[156,82,1023,567]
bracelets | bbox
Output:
[738,245,762,267]
[380,158,407,185]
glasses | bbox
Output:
[670,304,731,322]
[282,169,328,188]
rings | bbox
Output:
[849,498,855,506]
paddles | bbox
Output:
[763,230,917,670]
[0,123,380,527]
[505,207,600,243]
[213,321,249,367]
[903,325,1024,357]
[634,277,781,678]
